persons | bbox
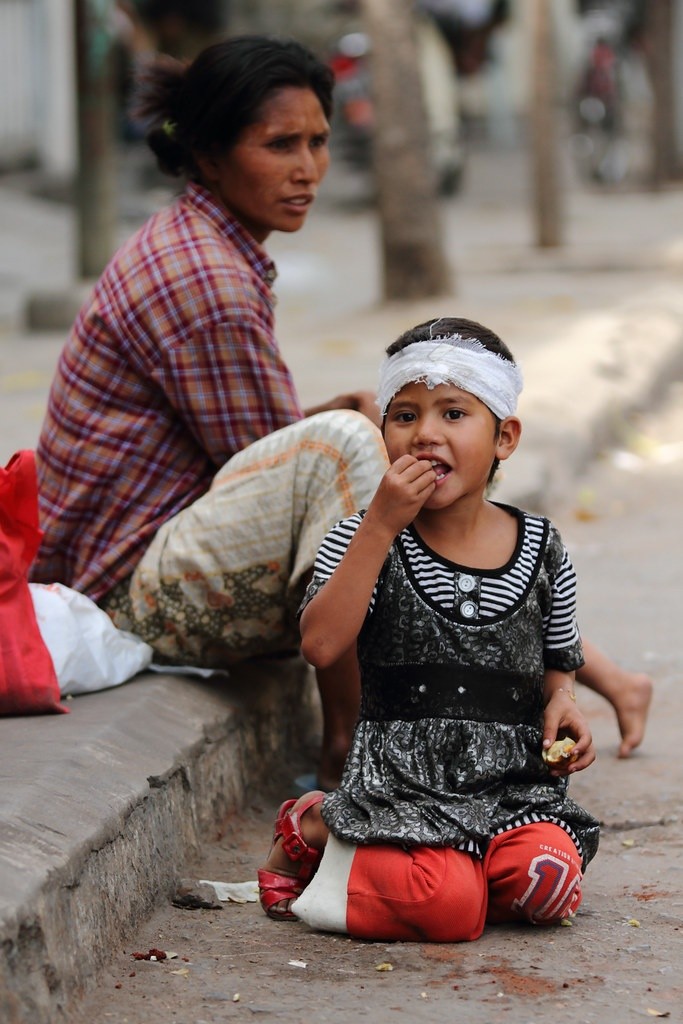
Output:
[259,317,600,941]
[573,638,654,759]
[36,36,383,791]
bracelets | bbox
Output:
[555,687,580,704]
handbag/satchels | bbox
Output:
[1,449,67,713]
[26,582,152,695]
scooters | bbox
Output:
[327,1,467,199]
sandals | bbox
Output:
[257,791,327,921]
